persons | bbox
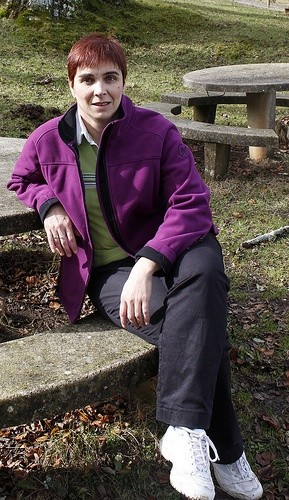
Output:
[7,34,264,500]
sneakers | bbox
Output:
[214,453,264,500]
[159,425,216,500]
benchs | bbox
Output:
[161,89,289,124]
[0,310,159,428]
[139,101,279,182]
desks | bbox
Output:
[183,63,289,159]
[1,137,45,237]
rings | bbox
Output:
[60,235,66,239]
[53,235,58,239]
